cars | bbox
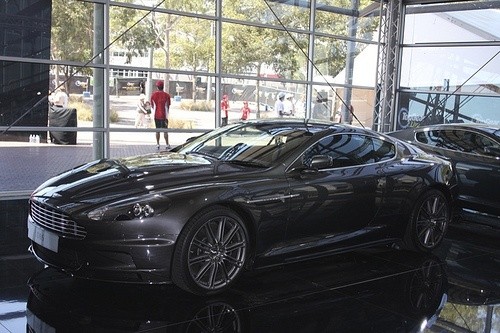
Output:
[447,208,500,306]
[26,241,449,333]
[27,118,461,297]
[383,123,500,217]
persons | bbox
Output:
[151,81,172,149]
[134,94,148,128]
[140,79,144,94]
[144,101,151,127]
[47,85,68,119]
[274,89,354,144]
[238,101,251,135]
[219,95,230,137]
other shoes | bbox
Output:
[166,146,170,149]
[157,147,160,150]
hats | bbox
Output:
[278,92,285,98]
[155,81,163,86]
